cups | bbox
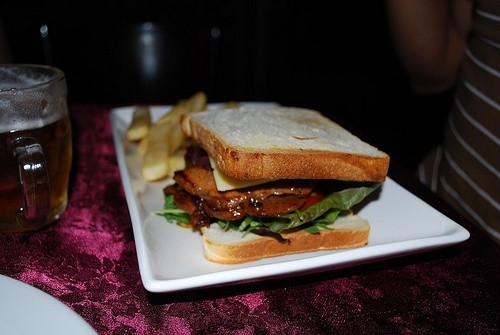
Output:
[0,64,73,234]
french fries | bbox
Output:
[120,92,204,180]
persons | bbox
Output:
[386,0,500,241]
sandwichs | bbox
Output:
[149,104,390,265]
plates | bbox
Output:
[0,274,96,335]
[109,101,470,293]
